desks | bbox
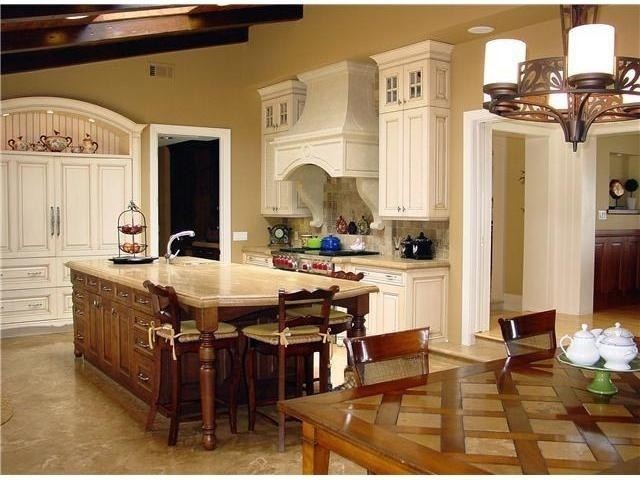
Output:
[275,335,640,475]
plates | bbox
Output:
[110,257,160,263]
[270,225,289,242]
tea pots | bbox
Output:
[559,323,604,367]
[8,129,98,154]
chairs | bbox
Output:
[276,271,364,398]
[143,279,245,447]
[498,309,557,357]
[241,285,339,453]
[343,326,430,475]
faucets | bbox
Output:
[167,230,196,262]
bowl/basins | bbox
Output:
[308,235,320,248]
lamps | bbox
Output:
[483,4,640,152]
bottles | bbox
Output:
[402,231,433,259]
[599,322,638,370]
[348,222,357,234]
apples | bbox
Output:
[123,244,140,251]
[122,226,140,234]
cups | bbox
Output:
[300,235,313,249]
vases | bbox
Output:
[627,197,636,210]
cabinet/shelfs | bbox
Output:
[86,274,134,395]
[134,288,157,408]
[70,268,86,361]
[370,40,455,222]
[0,151,134,331]
[256,79,313,218]
[351,264,449,344]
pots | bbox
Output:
[320,235,340,250]
